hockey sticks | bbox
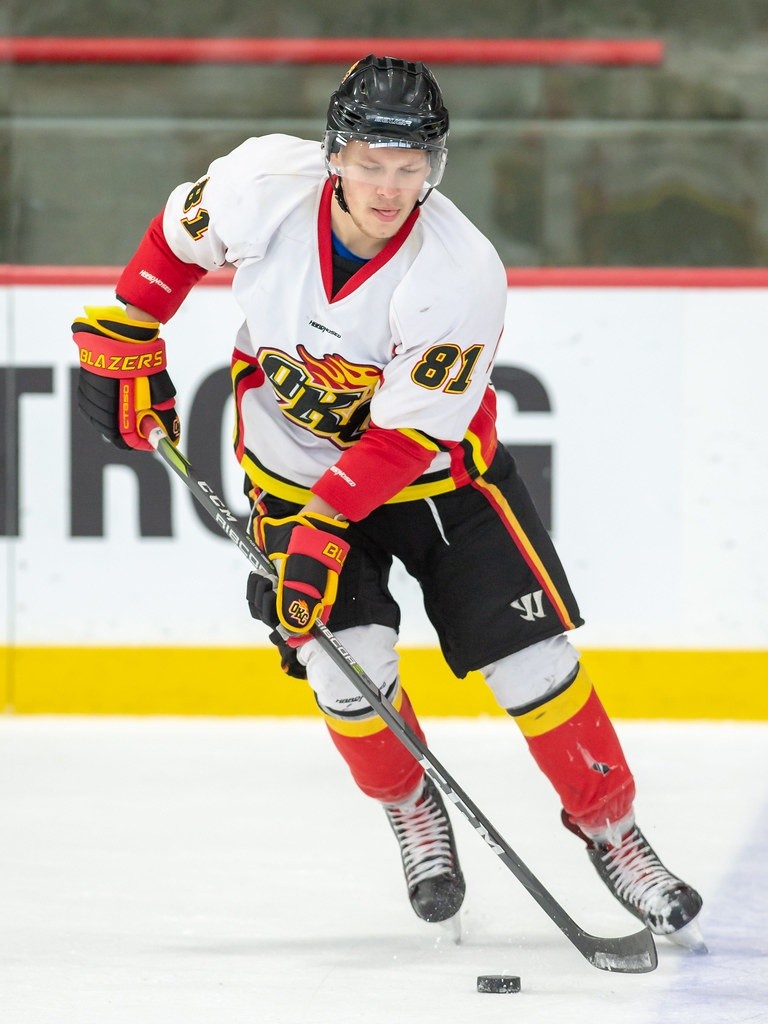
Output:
[138,416,660,973]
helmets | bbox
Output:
[327,53,450,159]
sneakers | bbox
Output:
[585,826,704,937]
[383,775,467,922]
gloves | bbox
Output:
[246,511,350,647]
[71,304,181,452]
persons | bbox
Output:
[66,50,702,955]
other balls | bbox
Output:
[477,976,523,994]
